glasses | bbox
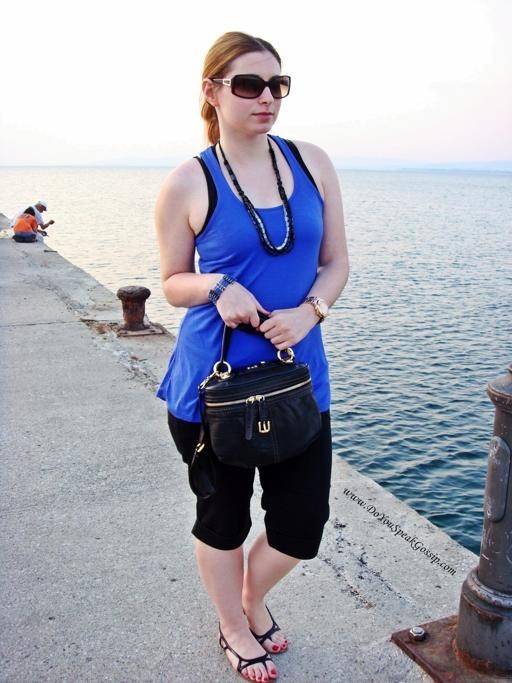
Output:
[209,73,293,102]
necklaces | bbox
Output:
[218,136,296,257]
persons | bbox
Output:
[152,30,350,683]
[12,207,40,243]
[10,199,56,236]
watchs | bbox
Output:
[301,294,330,324]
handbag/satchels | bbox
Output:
[199,307,323,471]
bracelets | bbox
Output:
[207,272,237,305]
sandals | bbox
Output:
[216,618,279,682]
[243,601,289,653]
